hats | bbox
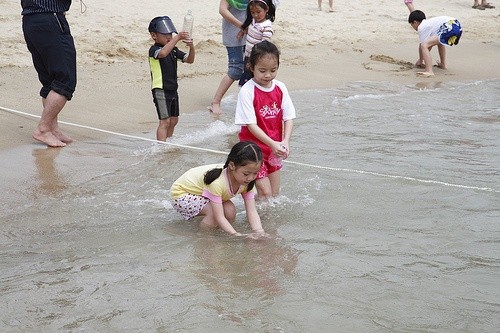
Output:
[149,15,179,34]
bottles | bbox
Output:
[182,10,194,42]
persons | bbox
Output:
[209,0,250,117]
[236,0,276,87]
[404,0,415,12]
[21,0,77,146]
[169,140,263,237]
[318,0,338,12]
[474,0,486,6]
[235,39,296,200]
[148,16,195,141]
[408,9,463,75]
